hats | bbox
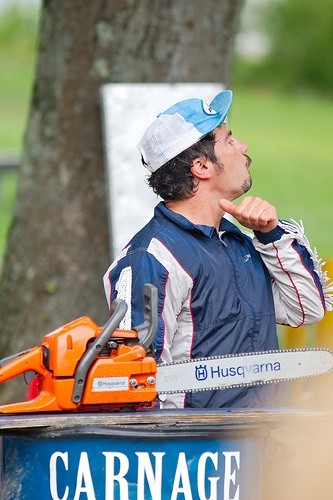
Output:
[136,89,233,174]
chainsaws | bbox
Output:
[0,283,333,416]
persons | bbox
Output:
[103,89,326,412]
